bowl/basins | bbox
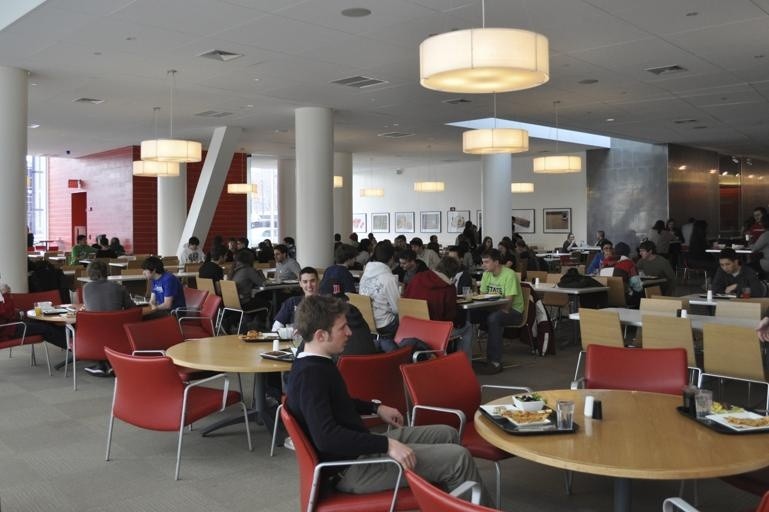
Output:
[277,328,294,339]
[512,396,545,414]
[732,244,744,249]
[37,301,52,310]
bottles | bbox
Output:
[677,308,688,317]
[584,395,603,419]
[272,339,281,352]
[706,290,713,299]
[745,233,754,242]
[532,277,540,284]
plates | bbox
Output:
[45,309,68,314]
[240,332,277,341]
[479,403,516,418]
[704,411,768,433]
[502,412,553,427]
[261,349,292,358]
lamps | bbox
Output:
[223,145,261,197]
[128,107,183,182]
[410,0,584,195]
[136,66,204,166]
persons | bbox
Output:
[283,292,500,510]
[264,265,377,452]
[318,205,769,374]
[0,227,302,376]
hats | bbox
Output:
[614,242,630,256]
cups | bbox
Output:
[681,383,698,412]
[695,390,712,417]
[462,286,470,299]
[713,242,718,249]
[33,302,42,318]
[718,244,725,249]
[556,399,575,430]
[742,286,751,299]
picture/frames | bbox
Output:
[351,207,581,238]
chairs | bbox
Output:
[404,470,504,512]
[566,343,697,498]
[574,307,631,385]
[266,338,412,460]
[525,240,768,350]
[0,244,531,408]
[697,323,769,405]
[396,350,574,508]
[660,491,769,512]
[125,315,245,433]
[101,343,256,483]
[640,312,701,386]
[279,407,421,512]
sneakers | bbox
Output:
[283,437,296,451]
[84,362,114,376]
[488,361,503,374]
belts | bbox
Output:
[330,469,347,487]
